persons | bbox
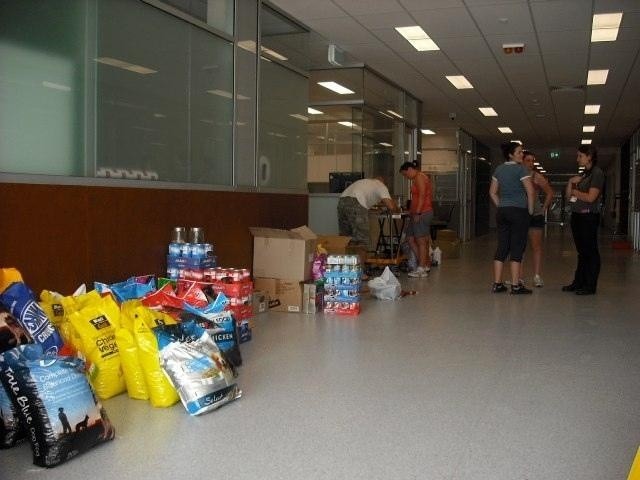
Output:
[489,144,533,299]
[516,150,554,285]
[333,173,398,283]
[560,143,604,297]
[400,161,435,280]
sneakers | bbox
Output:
[533,275,544,287]
[563,283,577,292]
[505,278,527,285]
[409,266,430,277]
[576,288,596,296]
[510,285,532,295]
[491,282,508,293]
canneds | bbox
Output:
[167,226,254,344]
[323,254,361,317]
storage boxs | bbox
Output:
[317,236,368,266]
[248,224,317,281]
[437,229,456,241]
[435,239,461,259]
[254,279,306,312]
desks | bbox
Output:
[367,209,410,277]
